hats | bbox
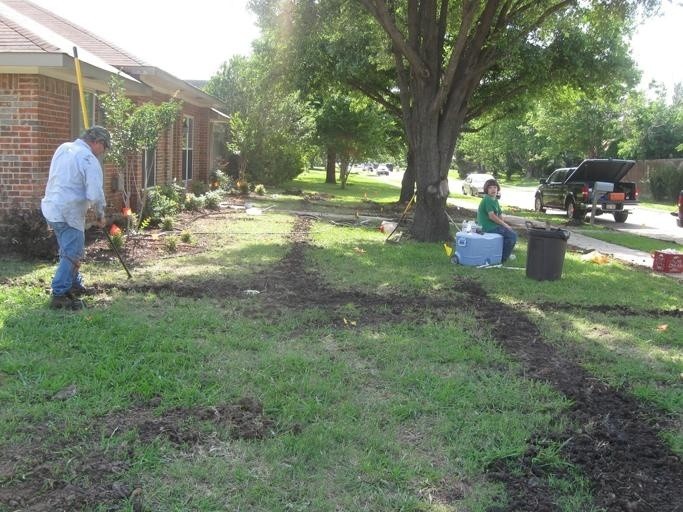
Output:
[83,126,111,149]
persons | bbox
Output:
[477,178,517,262]
[40,124,112,310]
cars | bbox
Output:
[462,174,496,197]
[376,163,391,176]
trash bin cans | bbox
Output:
[525,221,570,282]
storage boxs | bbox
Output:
[653,250,683,273]
[608,192,625,201]
[456,232,504,266]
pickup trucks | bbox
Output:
[535,168,640,222]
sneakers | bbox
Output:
[73,285,95,295]
[50,294,85,309]
